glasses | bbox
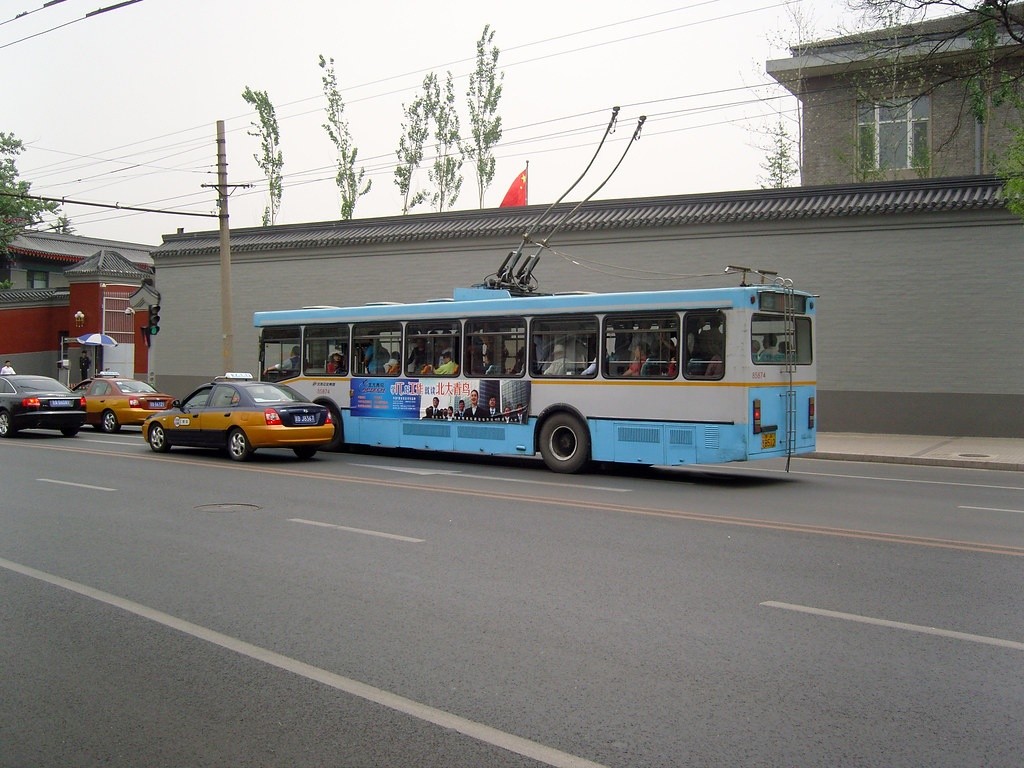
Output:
[443,356,449,359]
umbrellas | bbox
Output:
[77,333,119,374]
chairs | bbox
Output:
[485,365,501,375]
[641,358,666,375]
[686,358,705,374]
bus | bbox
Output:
[252,283,817,475]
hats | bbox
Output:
[385,359,398,367]
[82,350,87,353]
[552,344,564,352]
[440,349,452,355]
[328,349,344,356]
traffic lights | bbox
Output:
[149,304,161,335]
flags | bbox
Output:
[498,167,528,207]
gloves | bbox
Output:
[87,369,89,371]
[80,369,82,371]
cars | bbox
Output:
[0,374,87,438]
[142,372,335,462]
[71,371,175,433]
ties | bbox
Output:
[434,409,436,414]
[460,411,463,416]
[491,409,494,415]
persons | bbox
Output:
[610,318,723,376]
[475,352,494,374]
[543,344,575,375]
[263,345,300,376]
[434,351,459,375]
[383,351,400,375]
[364,331,390,374]
[0,361,17,374]
[759,334,779,362]
[751,339,761,360]
[327,350,345,373]
[80,350,90,382]
[778,342,792,355]
[581,357,596,375]
[429,323,596,373]
[423,389,524,423]
[622,343,650,376]
[407,338,426,372]
